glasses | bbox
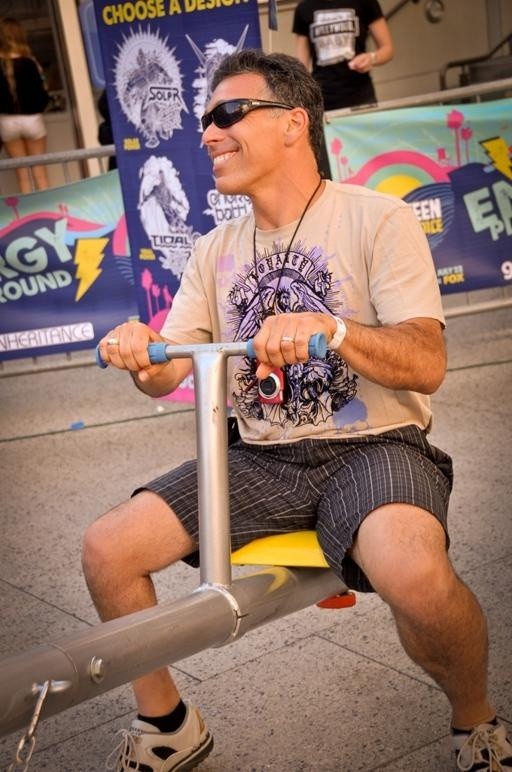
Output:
[202,96,296,128]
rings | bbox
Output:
[106,338,121,346]
[280,334,295,343]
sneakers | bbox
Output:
[113,707,214,772]
[448,717,511,772]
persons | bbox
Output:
[78,46,512,771]
[292,0,396,110]
[0,17,51,194]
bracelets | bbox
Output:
[327,313,347,351]
[370,52,377,66]
